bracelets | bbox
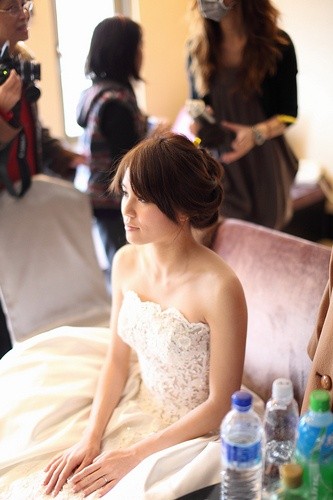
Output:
[0,108,14,120]
[264,119,272,140]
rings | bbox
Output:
[102,476,108,483]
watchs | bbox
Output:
[250,127,264,147]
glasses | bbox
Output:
[0,0,33,17]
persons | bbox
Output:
[0,1,298,288]
[0,131,277,500]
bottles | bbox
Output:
[272,461,311,500]
[290,388,333,500]
[220,389,266,500]
[261,378,300,500]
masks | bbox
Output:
[197,0,235,22]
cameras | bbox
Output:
[0,43,43,102]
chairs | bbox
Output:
[0,176,112,342]
[212,219,330,408]
